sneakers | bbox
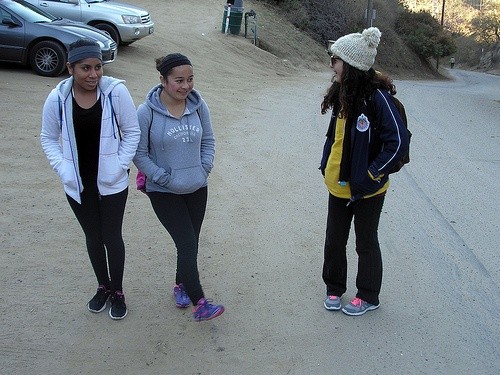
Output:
[342,298,381,315]
[323,296,341,310]
[88,284,110,313]
[174,283,190,308]
[191,298,224,322]
[109,290,128,319]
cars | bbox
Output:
[24,0,154,48]
[0,0,118,78]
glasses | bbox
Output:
[330,55,341,65]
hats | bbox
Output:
[330,27,381,71]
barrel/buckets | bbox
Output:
[222,4,244,35]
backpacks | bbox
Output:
[364,86,412,174]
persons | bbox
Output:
[40,39,141,319]
[318,26,409,315]
[132,53,226,321]
